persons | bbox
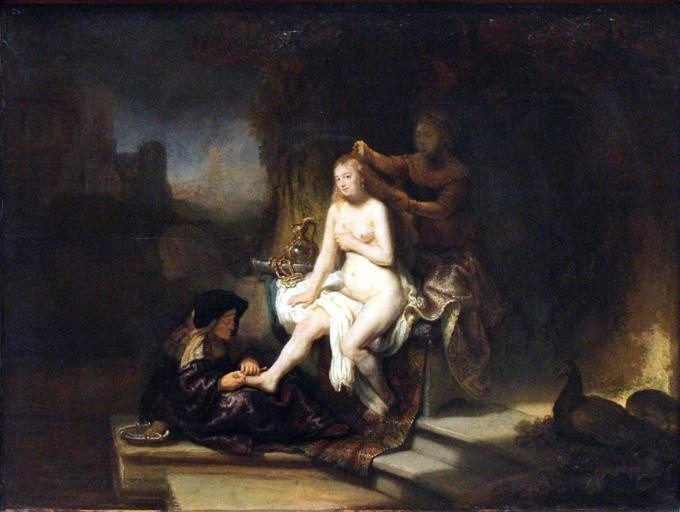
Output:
[352,114,515,404]
[241,149,407,414]
[139,286,351,459]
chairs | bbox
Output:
[244,254,493,415]
